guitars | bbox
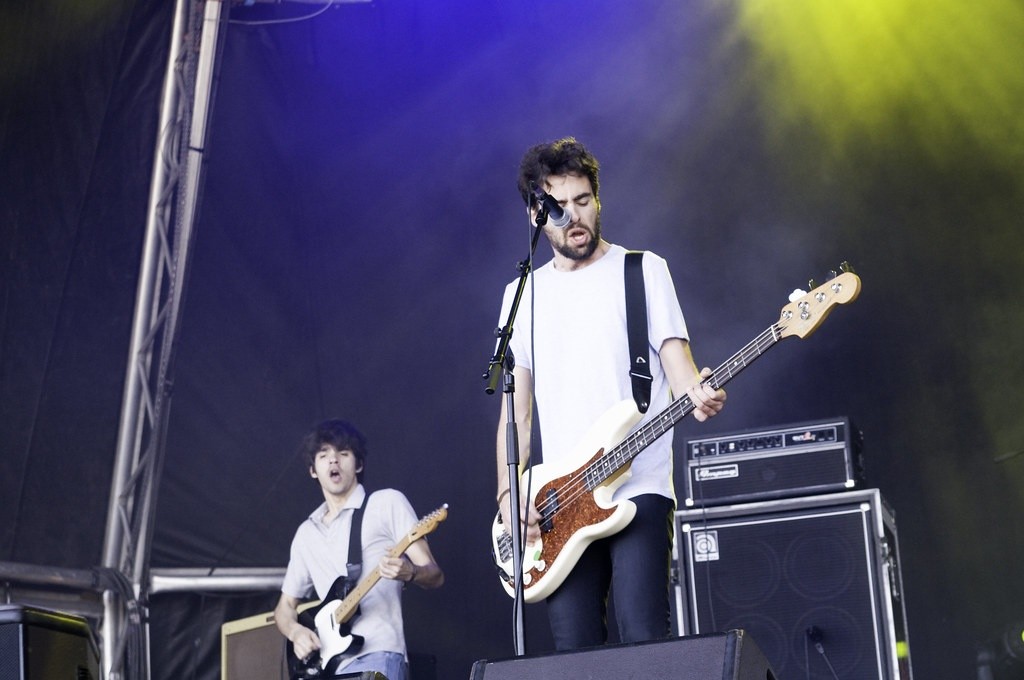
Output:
[286,506,447,680]
[491,262,861,603]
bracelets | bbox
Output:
[497,488,510,507]
[409,561,418,582]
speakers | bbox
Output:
[674,488,914,680]
[468,628,777,680]
[0,604,102,680]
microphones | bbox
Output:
[531,181,572,228]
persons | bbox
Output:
[274,421,444,680]
[490,136,730,657]
